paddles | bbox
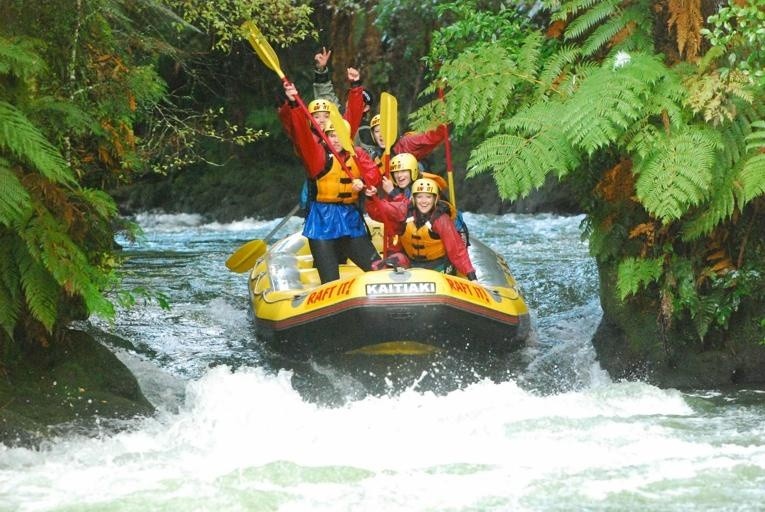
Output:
[224,203,300,273]
[239,21,355,181]
[379,92,397,269]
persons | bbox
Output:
[280,45,383,285]
[362,113,477,282]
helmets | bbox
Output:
[307,97,332,128]
[322,118,352,145]
[370,114,382,146]
[348,87,373,106]
[388,153,419,188]
[411,178,438,208]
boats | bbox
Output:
[248,217,532,362]
